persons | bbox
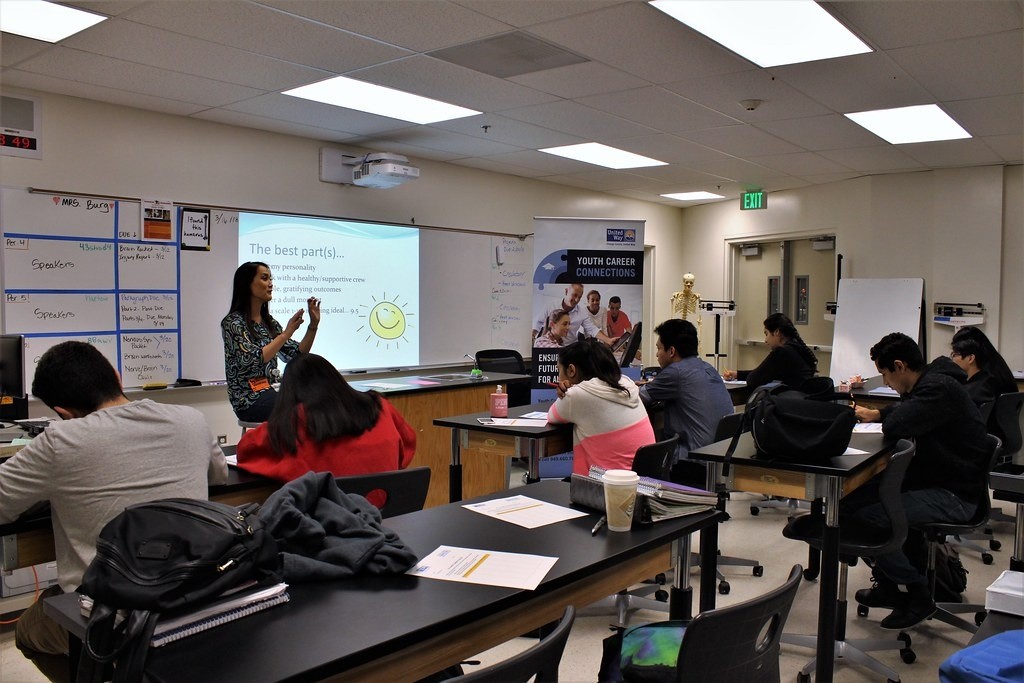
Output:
[237,354,416,483]
[950,326,1017,423]
[547,338,656,483]
[723,313,818,395]
[532,283,633,352]
[0,340,229,683]
[848,333,988,629]
[221,261,321,422]
[640,319,734,489]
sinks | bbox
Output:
[418,374,470,382]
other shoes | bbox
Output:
[855,578,907,609]
[879,595,937,631]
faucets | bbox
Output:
[463,354,476,369]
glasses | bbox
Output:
[951,351,966,358]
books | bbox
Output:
[570,464,719,523]
[79,581,290,647]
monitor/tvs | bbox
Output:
[620,321,642,368]
[0,335,26,443]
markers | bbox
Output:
[349,369,367,373]
[390,368,401,371]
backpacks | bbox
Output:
[722,378,856,461]
[77,496,274,666]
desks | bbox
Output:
[339,373,533,509]
[433,399,560,506]
[687,422,901,683]
[831,372,902,403]
[1,446,244,547]
[43,476,727,683]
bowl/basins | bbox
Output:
[850,379,868,388]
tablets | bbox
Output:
[610,331,631,353]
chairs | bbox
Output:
[630,431,681,474]
[616,563,803,683]
[336,466,432,516]
[856,431,1003,663]
[943,390,1024,564]
[441,603,577,683]
[477,347,525,374]
[778,438,918,683]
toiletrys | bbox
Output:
[490,384,508,418]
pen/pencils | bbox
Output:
[849,390,861,424]
[723,367,734,380]
[592,515,606,534]
[545,382,559,386]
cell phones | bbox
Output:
[477,418,495,424]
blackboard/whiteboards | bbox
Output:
[830,276,924,387]
[1,186,531,400]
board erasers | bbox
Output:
[496,245,505,265]
[174,378,202,387]
[143,382,168,390]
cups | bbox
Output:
[603,470,640,532]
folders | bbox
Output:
[570,473,716,525]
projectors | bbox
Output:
[352,164,419,189]
